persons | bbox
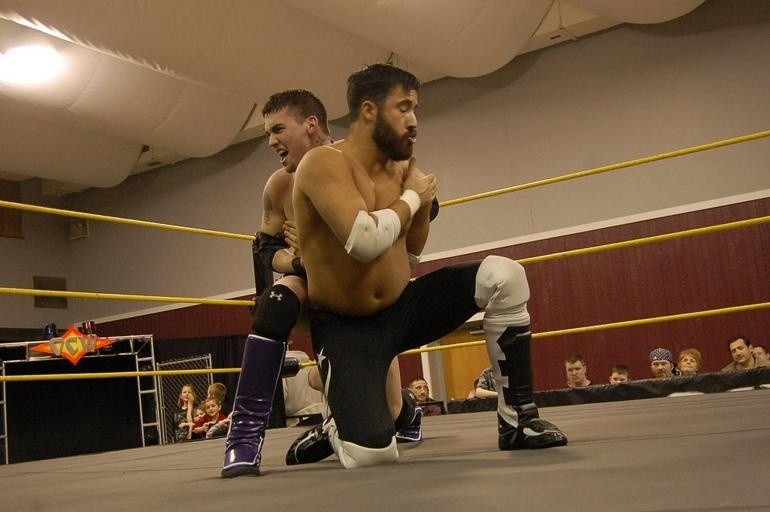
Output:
[284,63,568,467]
[190,404,208,424]
[564,355,599,389]
[281,350,324,429]
[475,367,498,400]
[752,343,770,364]
[221,89,440,478]
[677,347,705,375]
[193,396,226,441]
[406,377,442,417]
[648,347,678,379]
[173,382,199,444]
[721,333,770,376]
[607,364,632,386]
[200,382,230,419]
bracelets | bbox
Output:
[399,188,421,219]
[292,255,304,274]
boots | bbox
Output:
[482,323,568,450]
[285,414,338,464]
[395,390,423,442]
[220,334,287,477]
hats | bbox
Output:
[649,348,673,362]
